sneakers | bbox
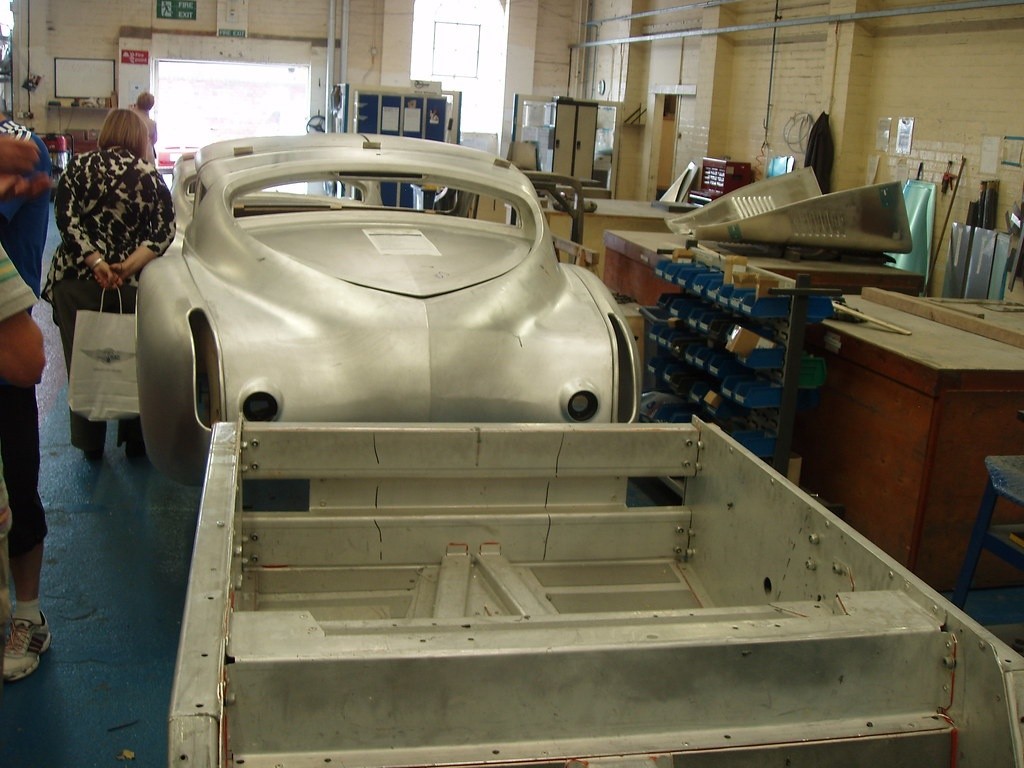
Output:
[3,609,52,680]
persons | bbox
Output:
[0,114,52,682]
[41,109,176,461]
[134,93,157,167]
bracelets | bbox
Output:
[90,258,103,271]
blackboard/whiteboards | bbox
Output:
[53,56,116,99]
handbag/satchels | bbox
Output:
[68,287,139,422]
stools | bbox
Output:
[950,455,1024,612]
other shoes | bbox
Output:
[82,447,104,459]
[126,441,146,458]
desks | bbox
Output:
[802,296,1024,589]
[602,229,925,308]
